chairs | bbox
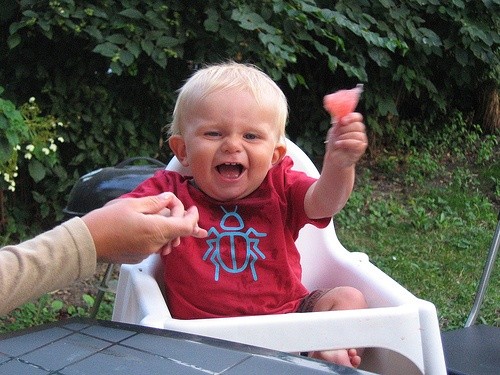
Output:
[110,137,447,375]
[441,210,499,375]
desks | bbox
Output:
[0,317,383,375]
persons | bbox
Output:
[104,58,369,368]
[0,191,199,317]
